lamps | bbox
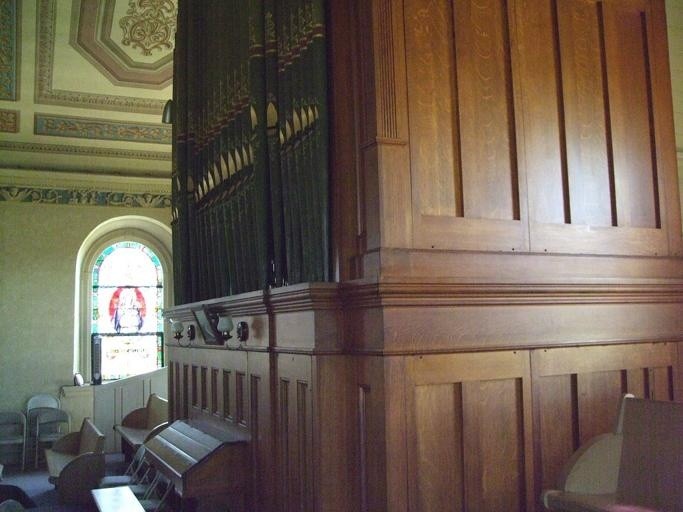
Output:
[171,321,195,348]
[217,314,249,351]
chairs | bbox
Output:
[98,445,176,512]
[0,392,72,473]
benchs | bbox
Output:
[44,416,106,505]
[539,393,682,512]
[113,394,170,461]
